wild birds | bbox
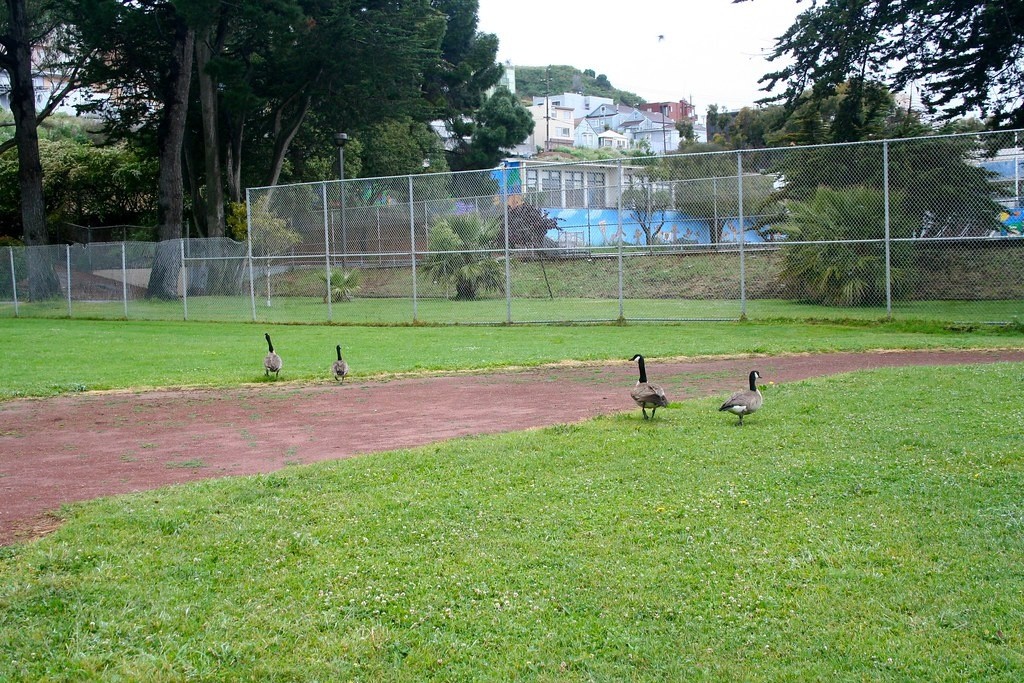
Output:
[263,333,282,378]
[332,344,348,384]
[629,354,668,420]
[720,371,763,425]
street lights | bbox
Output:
[334,132,349,274]
